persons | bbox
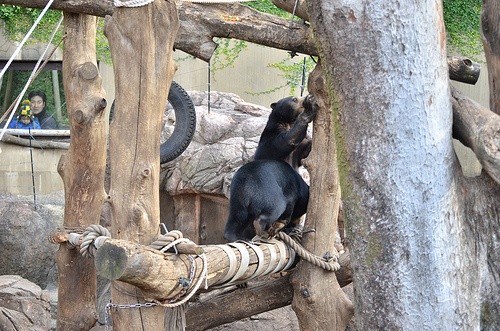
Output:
[7,100,41,130]
[28,89,58,129]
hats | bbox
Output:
[17,99,34,122]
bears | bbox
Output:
[254,92,319,170]
[223,159,310,244]
